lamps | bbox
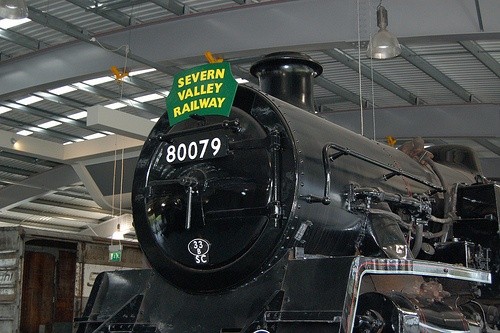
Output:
[365,0,402,61]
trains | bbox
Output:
[80,53,500,333]
